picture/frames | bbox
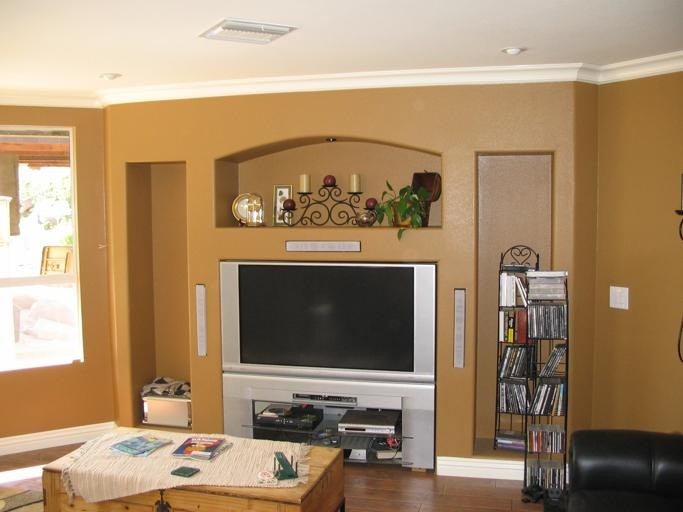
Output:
[271,184,292,227]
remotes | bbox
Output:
[275,451,296,476]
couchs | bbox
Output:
[560,429,683,511]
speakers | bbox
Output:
[196,285,207,357]
[453,288,466,368]
[285,240,361,253]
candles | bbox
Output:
[348,174,361,192]
[299,174,311,193]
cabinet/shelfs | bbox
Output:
[494,245,539,450]
[521,276,569,503]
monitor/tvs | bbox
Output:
[219,261,437,383]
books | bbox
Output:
[170,433,224,457]
[492,266,566,494]
[130,444,158,457]
[108,432,170,455]
[188,438,233,461]
